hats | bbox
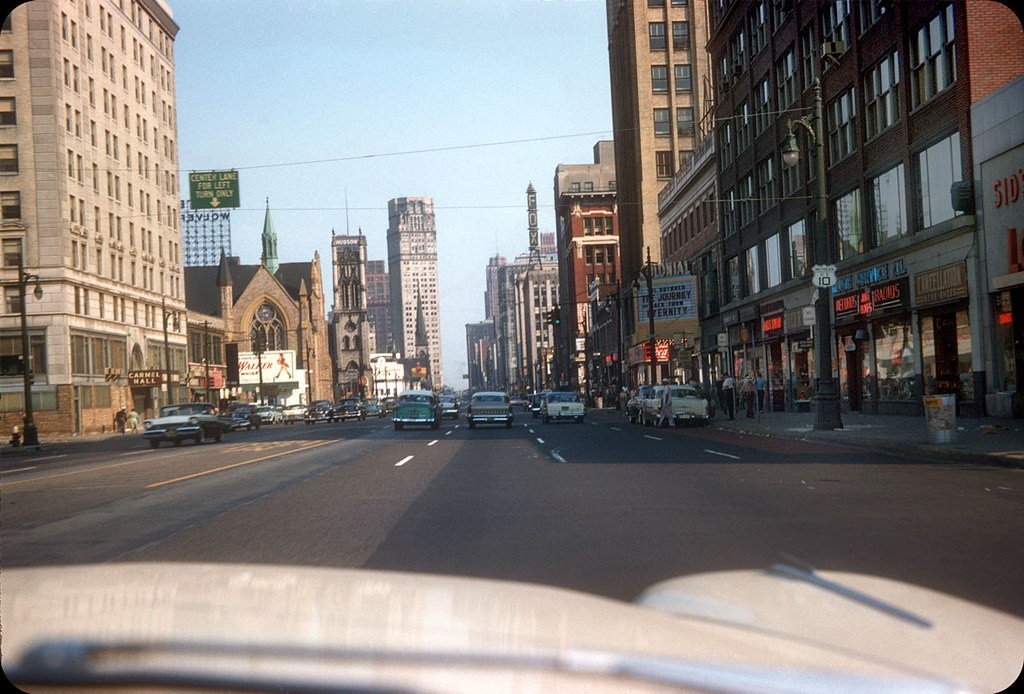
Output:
[663,378,668,382]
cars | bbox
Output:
[142,403,232,449]
[304,400,335,425]
[509,389,587,423]
[361,397,397,419]
[332,397,367,423]
[221,402,261,432]
[391,390,442,430]
[439,393,470,420]
[625,384,711,427]
[256,404,307,426]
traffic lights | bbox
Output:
[251,342,264,355]
[595,362,601,369]
[645,341,655,357]
[546,308,561,326]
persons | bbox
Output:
[654,378,676,429]
[722,372,734,421]
[275,354,292,379]
[127,408,140,434]
[800,363,809,387]
[694,385,712,427]
[715,375,727,415]
[114,407,127,435]
[739,373,756,418]
[754,373,766,414]
[611,376,623,411]
[792,371,797,400]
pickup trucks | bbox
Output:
[466,392,515,429]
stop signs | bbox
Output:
[356,376,368,386]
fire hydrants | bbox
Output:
[9,426,21,446]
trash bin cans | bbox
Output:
[922,393,957,445]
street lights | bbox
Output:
[373,365,380,398]
[161,298,179,406]
[384,366,390,397]
[634,246,657,386]
[605,279,622,410]
[393,372,421,397]
[521,327,570,394]
[17,252,43,446]
[305,340,316,403]
[778,77,843,429]
[575,311,592,408]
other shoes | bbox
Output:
[760,410,764,414]
